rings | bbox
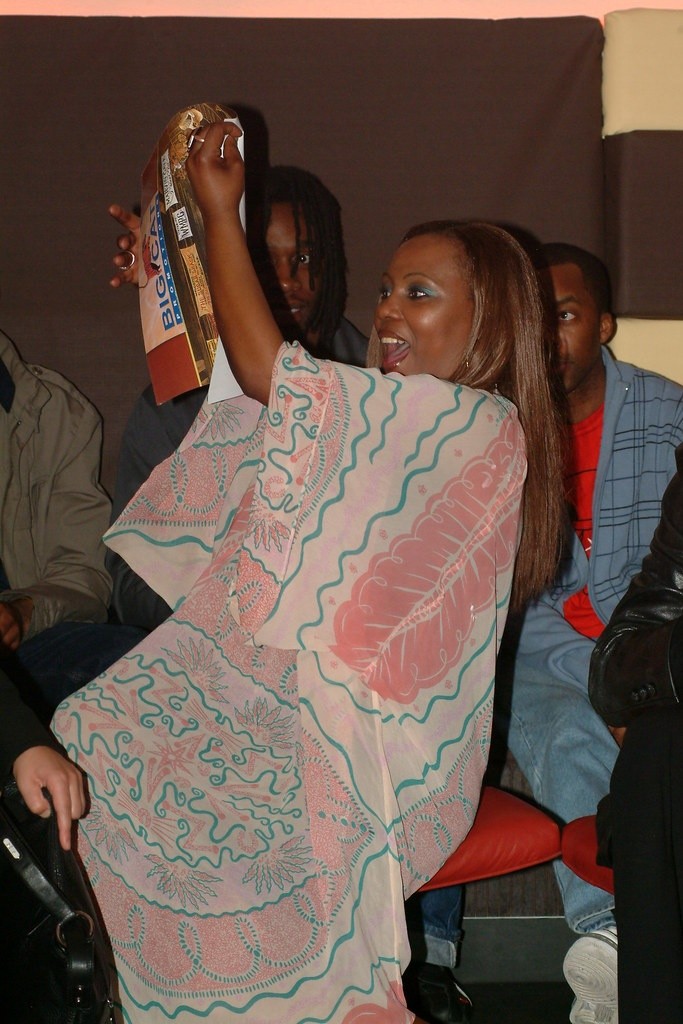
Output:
[195,133,205,143]
[120,248,137,270]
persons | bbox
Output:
[100,160,481,1024]
[57,116,573,1024]
[0,325,135,1024]
[588,439,683,1023]
[496,239,683,1024]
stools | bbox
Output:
[400,786,562,1024]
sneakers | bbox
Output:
[402,960,475,1024]
[563,925,617,1023]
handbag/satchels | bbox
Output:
[0,780,109,1023]
